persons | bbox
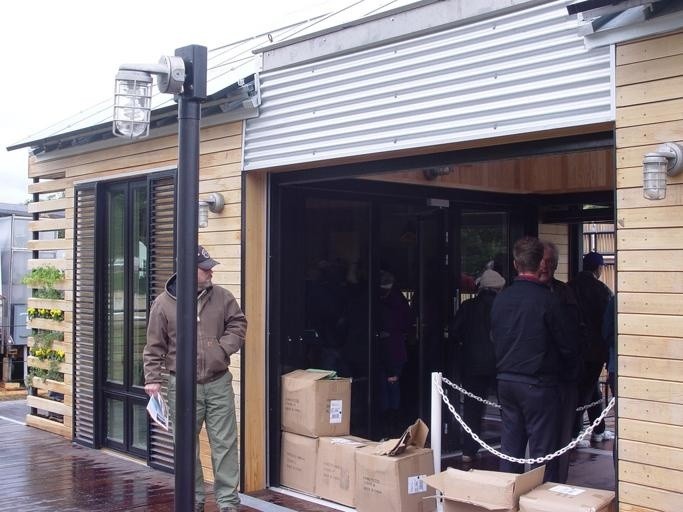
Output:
[532,237,593,485]
[599,291,615,421]
[447,266,506,464]
[486,232,586,485]
[141,244,249,512]
[302,255,354,371]
[359,268,417,427]
[562,251,614,451]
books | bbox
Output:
[145,392,169,432]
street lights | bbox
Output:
[110,44,207,512]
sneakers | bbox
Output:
[193,503,237,512]
[572,437,591,448]
[593,430,615,442]
[462,452,481,462]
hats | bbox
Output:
[583,252,607,266]
[479,268,505,287]
[197,246,221,271]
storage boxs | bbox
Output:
[317,436,379,510]
[355,440,437,512]
[519,480,617,511]
[422,464,546,511]
[279,431,317,500]
[282,368,352,439]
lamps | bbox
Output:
[196,192,224,227]
[642,141,683,200]
[423,165,454,181]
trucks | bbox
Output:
[0,203,65,388]
